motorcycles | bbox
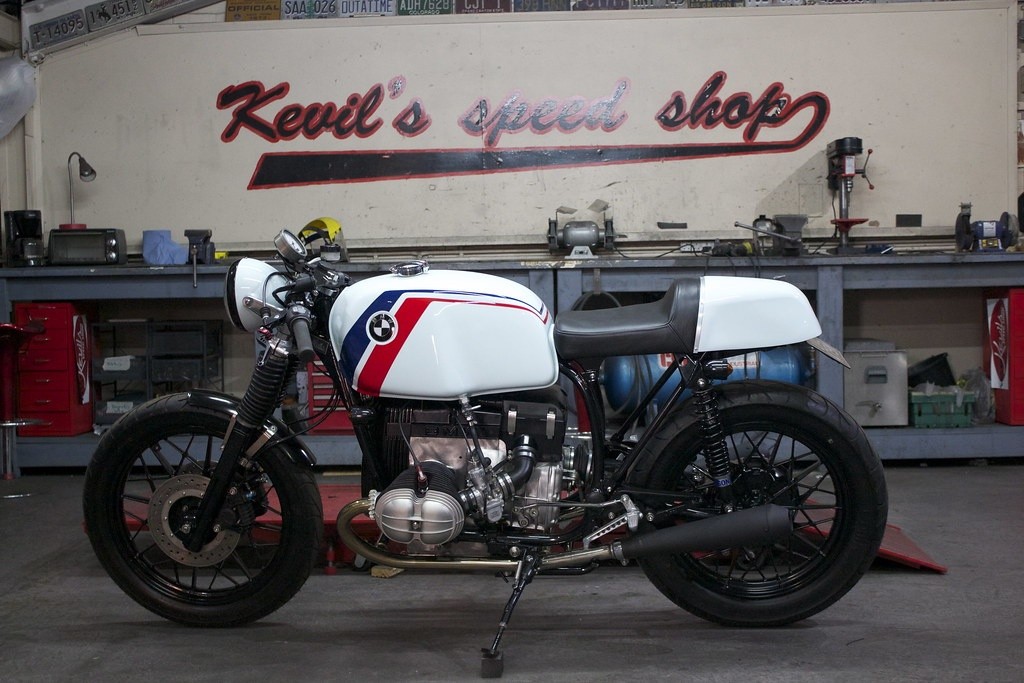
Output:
[80,229,889,678]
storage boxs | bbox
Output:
[842,349,908,427]
[909,391,977,430]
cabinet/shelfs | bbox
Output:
[10,301,94,438]
[107,315,225,423]
